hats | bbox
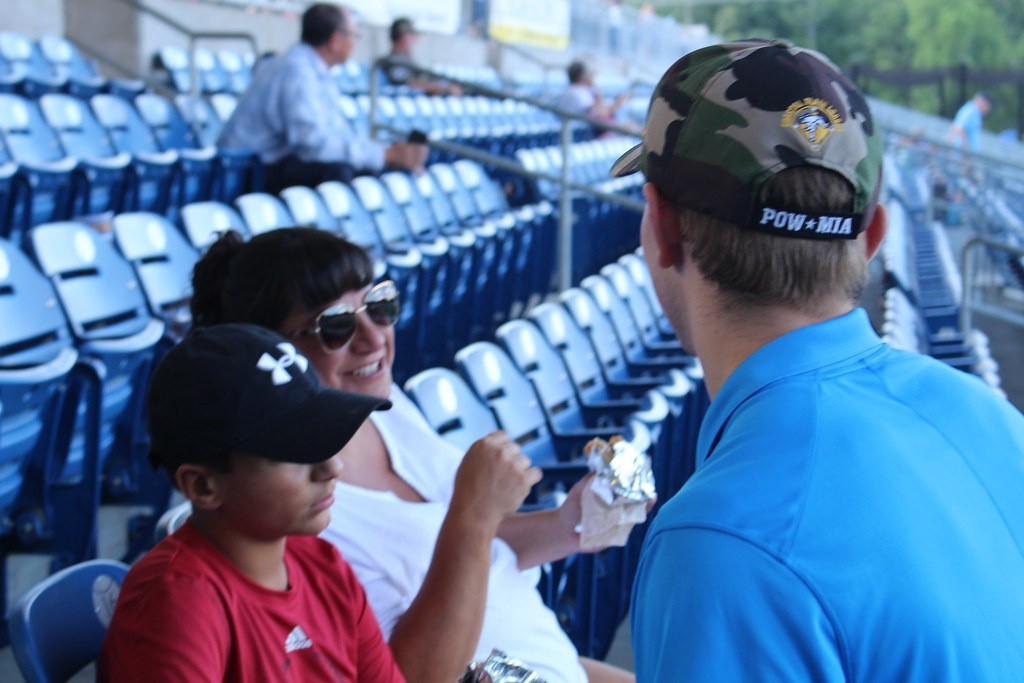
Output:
[610,38,883,241]
[147,323,393,474]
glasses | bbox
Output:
[286,281,400,354]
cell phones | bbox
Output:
[407,131,426,145]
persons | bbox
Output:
[99,322,409,683]
[372,19,462,94]
[559,62,628,119]
[189,227,658,683]
[612,39,1021,683]
[218,2,430,172]
[949,89,993,153]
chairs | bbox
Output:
[0,33,1024,683]
